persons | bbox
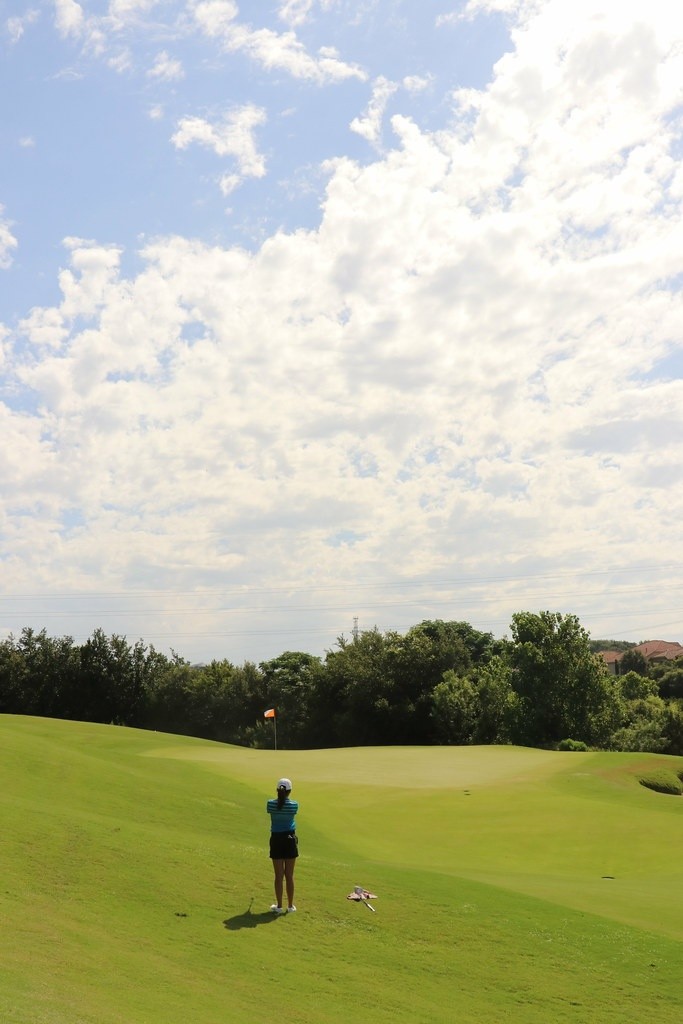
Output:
[267,778,299,912]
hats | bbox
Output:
[277,778,292,791]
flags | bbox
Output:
[264,709,274,717]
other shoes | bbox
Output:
[287,905,297,912]
[270,905,283,913]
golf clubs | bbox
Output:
[354,886,375,912]
